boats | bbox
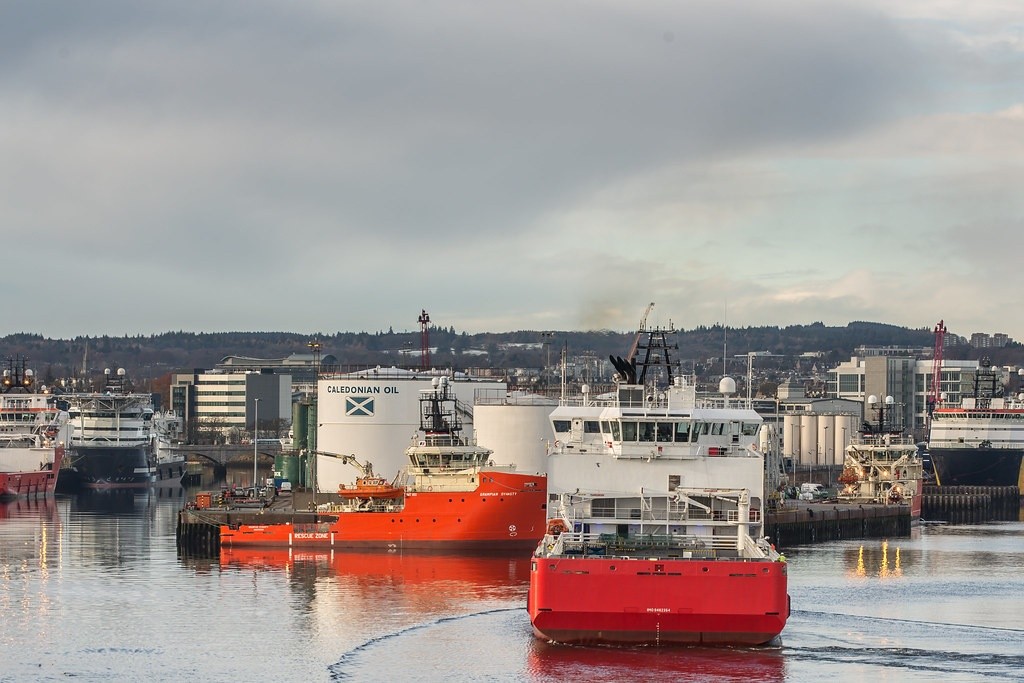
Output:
[336,461,405,500]
[923,354,1024,496]
[837,392,922,526]
[50,367,186,488]
[0,352,73,495]
[527,300,791,647]
[219,376,550,552]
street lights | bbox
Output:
[539,331,556,388]
[842,427,847,471]
[824,426,828,466]
[795,423,806,464]
[254,399,262,498]
[828,448,832,488]
[792,449,800,488]
[307,340,320,397]
[808,449,815,484]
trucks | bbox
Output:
[801,483,828,494]
